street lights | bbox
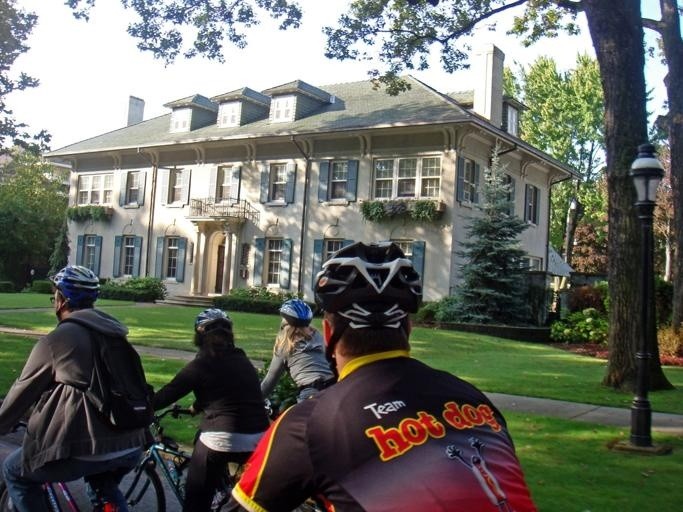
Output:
[621,143,665,448]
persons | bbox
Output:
[0,263,156,511]
[259,297,335,403]
[152,307,271,512]
[220,239,536,512]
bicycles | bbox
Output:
[0,391,323,511]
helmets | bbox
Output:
[312,238,422,321]
[46,265,102,310]
[193,307,233,333]
[278,298,312,329]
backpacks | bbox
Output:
[54,316,156,430]
